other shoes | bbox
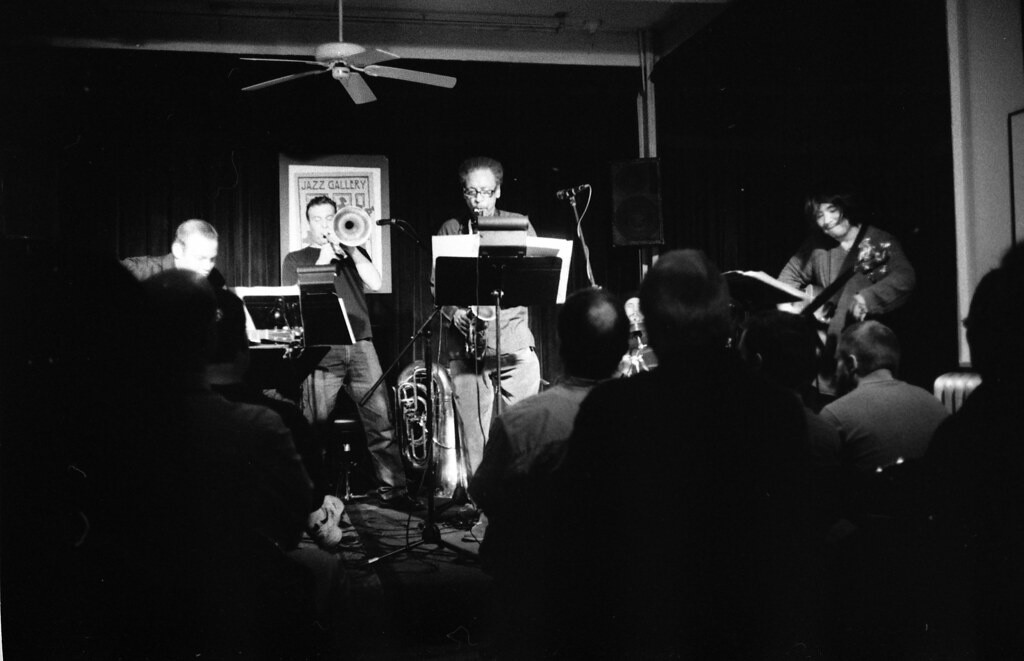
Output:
[378,492,426,511]
[425,495,480,521]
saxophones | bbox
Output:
[465,210,491,365]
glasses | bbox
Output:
[462,183,497,200]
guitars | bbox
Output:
[244,320,306,348]
[797,244,890,315]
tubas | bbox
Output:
[394,360,469,499]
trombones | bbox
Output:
[322,204,374,258]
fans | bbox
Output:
[238,0,457,105]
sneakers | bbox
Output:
[307,494,345,552]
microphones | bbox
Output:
[376,219,404,226]
[557,184,589,200]
[471,207,479,233]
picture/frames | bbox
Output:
[277,150,393,293]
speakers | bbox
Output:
[610,158,665,246]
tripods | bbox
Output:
[358,305,479,567]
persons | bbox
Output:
[120,219,263,344]
[282,196,425,511]
[430,158,541,514]
[466,238,1024,661]
[140,271,346,661]
[777,187,915,407]
